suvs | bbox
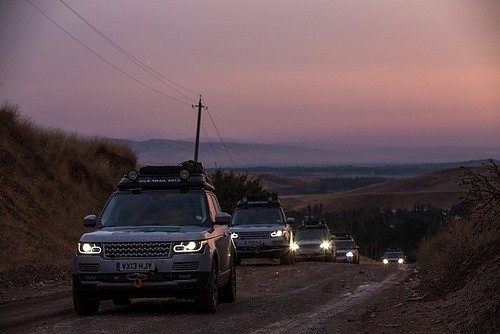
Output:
[293,218,336,263]
[381,248,407,265]
[229,192,295,266]
[70,159,237,312]
[330,233,361,264]
